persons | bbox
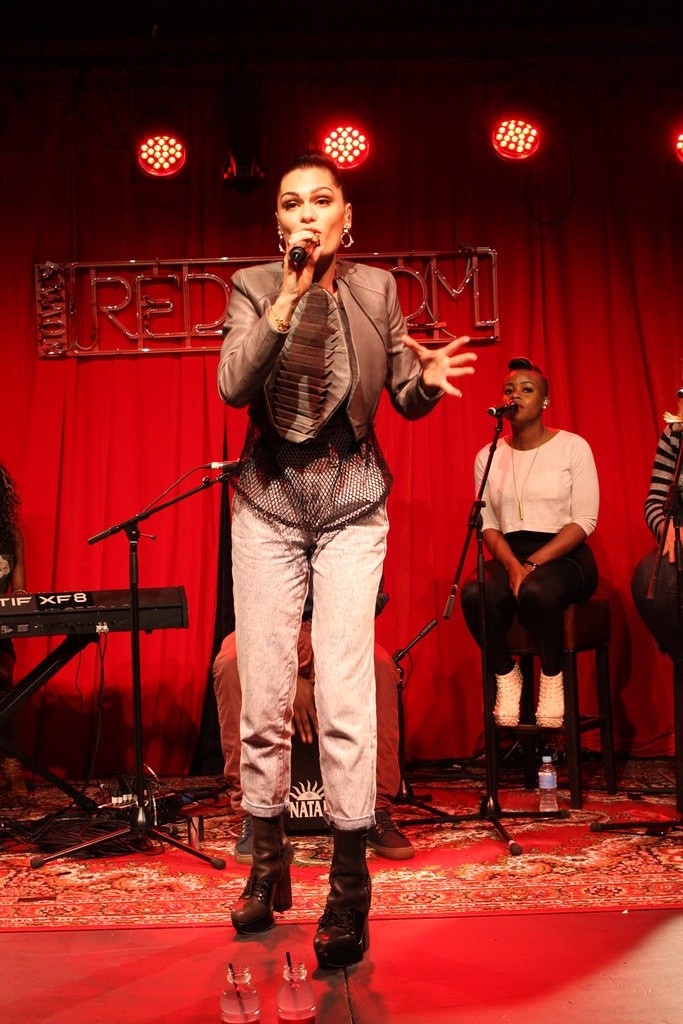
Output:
[644,388,683,563]
[212,545,414,859]
[218,149,475,969]
[0,462,28,810]
[459,359,599,728]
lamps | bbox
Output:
[127,105,193,186]
[312,89,376,174]
[664,96,683,167]
[486,87,550,165]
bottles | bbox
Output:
[538,755,558,811]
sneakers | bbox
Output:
[234,814,253,864]
[365,812,416,859]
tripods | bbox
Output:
[391,413,570,855]
[30,470,237,871]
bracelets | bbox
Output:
[523,560,538,571]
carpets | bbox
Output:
[0,758,683,934]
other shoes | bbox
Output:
[492,660,523,727]
[535,668,565,728]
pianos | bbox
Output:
[0,583,192,815]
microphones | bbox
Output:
[676,389,683,398]
[288,239,314,264]
[487,401,518,417]
[205,462,238,471]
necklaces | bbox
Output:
[511,428,545,520]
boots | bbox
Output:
[230,818,292,936]
[313,827,372,969]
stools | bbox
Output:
[489,599,617,810]
